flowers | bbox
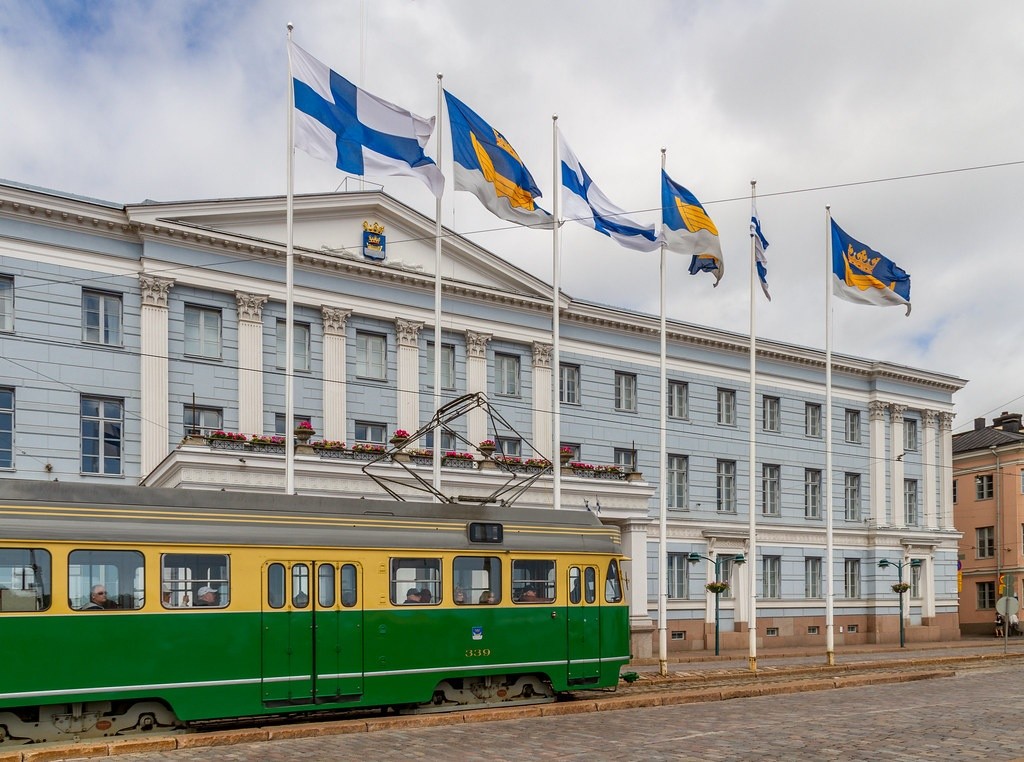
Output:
[705,580,729,592]
[207,419,620,474]
[891,582,912,591]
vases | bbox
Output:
[352,450,385,460]
[572,467,595,478]
[525,465,552,473]
[894,586,906,593]
[596,470,620,480]
[313,448,344,458]
[409,454,434,465]
[476,446,496,460]
[389,437,410,449]
[710,587,725,592]
[443,457,473,469]
[294,428,315,445]
[495,462,526,474]
[250,442,286,453]
[210,439,244,451]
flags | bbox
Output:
[556,125,662,252]
[286,37,445,201]
[661,168,724,288]
[749,206,771,302]
[442,88,566,230]
[831,217,912,317]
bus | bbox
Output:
[0,477,632,748]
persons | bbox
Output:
[518,585,549,601]
[453,584,495,605]
[79,584,107,610]
[193,586,217,606]
[163,584,189,608]
[403,588,432,603]
[994,612,1023,638]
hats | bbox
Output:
[523,585,540,593]
[406,588,423,596]
[162,584,171,593]
[196,587,217,597]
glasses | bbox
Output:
[91,590,107,596]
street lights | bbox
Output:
[877,558,921,647]
[686,552,745,655]
